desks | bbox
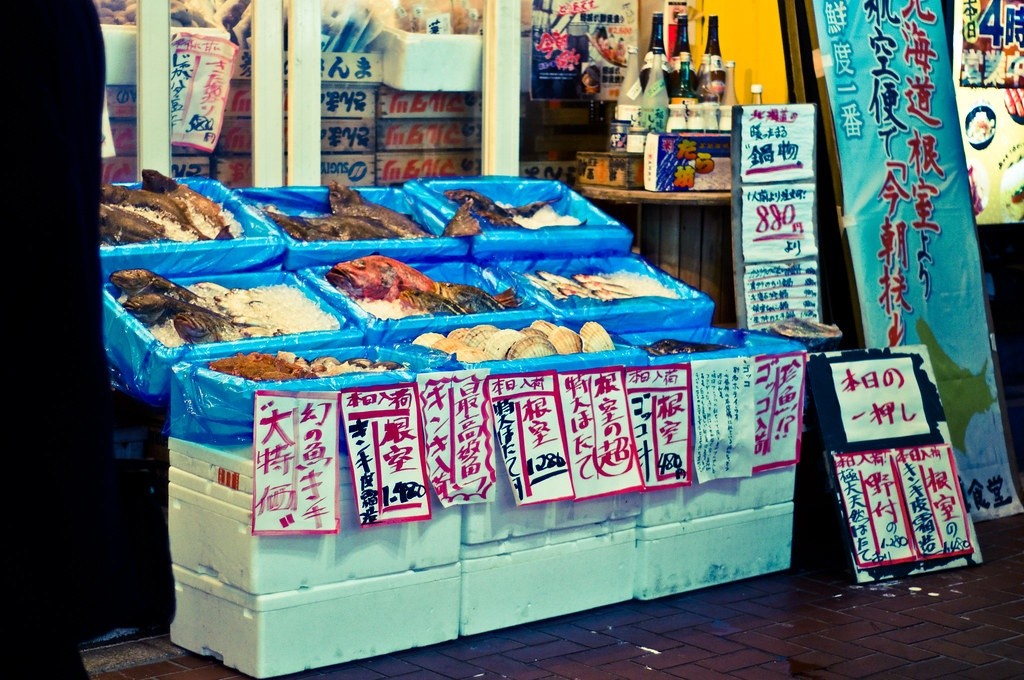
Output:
[581,184,733,324]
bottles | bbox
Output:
[641,47,669,134]
[667,104,686,133]
[751,84,763,105]
[721,61,741,104]
[702,103,719,133]
[719,106,732,133]
[671,52,698,104]
[697,16,726,102]
[670,13,698,90]
[695,54,718,104]
[608,45,643,127]
[687,103,704,133]
[641,11,670,93]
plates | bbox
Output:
[1003,100,1024,126]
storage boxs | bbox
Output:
[95,18,801,677]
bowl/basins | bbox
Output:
[965,104,996,150]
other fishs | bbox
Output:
[208,351,405,382]
[644,338,735,358]
[527,267,635,304]
[100,168,236,243]
[111,267,294,343]
[325,256,522,319]
[268,179,590,243]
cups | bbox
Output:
[567,22,589,63]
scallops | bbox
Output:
[412,320,616,362]
